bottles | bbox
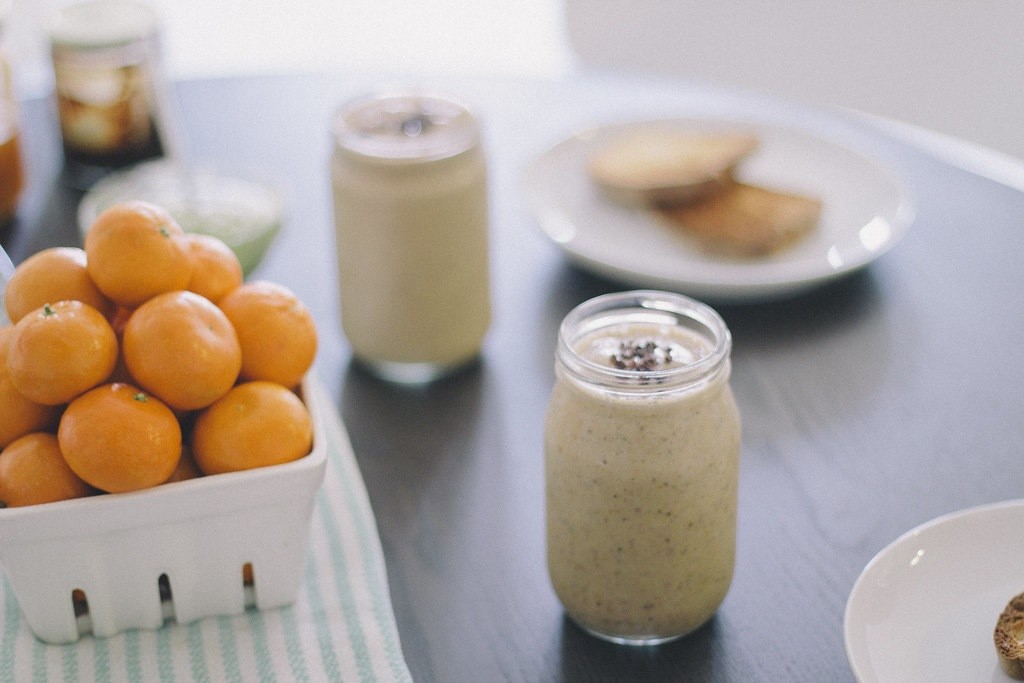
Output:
[47,2,159,184]
[327,93,490,384]
[1,61,27,225]
[544,290,744,647]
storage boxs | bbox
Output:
[0,372,326,650]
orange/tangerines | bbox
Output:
[0,201,317,510]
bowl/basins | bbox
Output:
[77,164,284,283]
[0,374,327,644]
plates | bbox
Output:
[526,114,917,297]
[842,499,1024,682]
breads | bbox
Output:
[588,125,821,258]
[993,589,1024,680]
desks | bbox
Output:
[0,77,1024,683]
